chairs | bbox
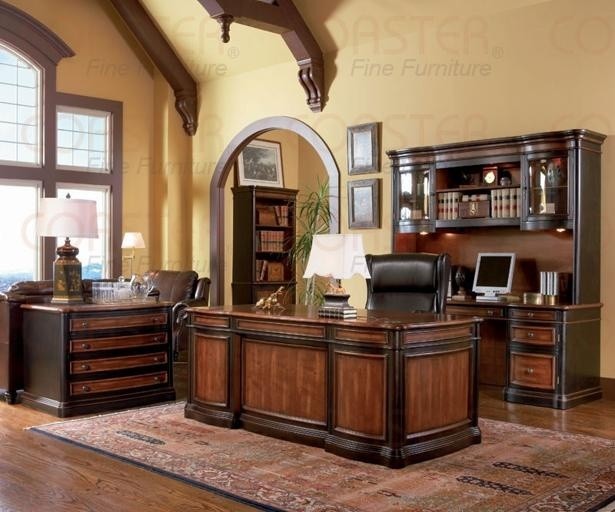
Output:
[1,278,100,405]
[142,269,212,362]
[364,252,452,315]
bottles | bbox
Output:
[536,159,567,187]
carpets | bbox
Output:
[21,394,615,512]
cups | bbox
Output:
[91,271,154,301]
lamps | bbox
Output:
[120,232,146,259]
[37,192,99,304]
[303,234,372,320]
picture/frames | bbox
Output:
[346,122,381,229]
[235,138,285,188]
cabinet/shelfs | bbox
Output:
[19,302,176,416]
[502,309,561,410]
[384,128,608,303]
[230,187,300,304]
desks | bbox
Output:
[447,299,603,411]
[184,303,485,470]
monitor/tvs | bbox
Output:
[472,253,516,301]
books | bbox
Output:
[318,312,357,318]
[318,306,356,314]
[540,270,568,296]
[435,187,522,220]
[255,203,289,281]
